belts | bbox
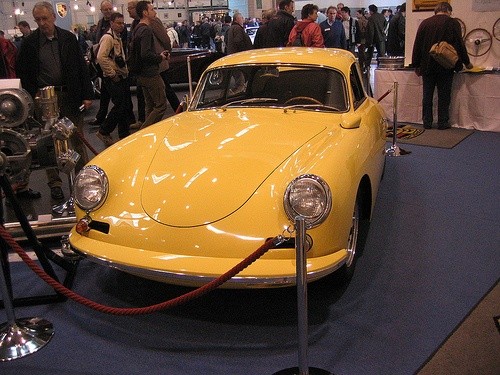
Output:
[36,85,72,92]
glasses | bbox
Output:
[34,13,54,23]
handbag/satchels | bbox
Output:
[173,40,179,48]
[214,35,222,42]
[429,41,459,69]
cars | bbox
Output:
[66,48,388,291]
[126,48,230,92]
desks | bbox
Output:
[375,68,500,133]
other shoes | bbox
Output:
[129,121,144,130]
[51,186,64,201]
[95,129,115,146]
[438,123,451,130]
[16,188,41,199]
[88,119,105,126]
[423,122,432,129]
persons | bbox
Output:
[254,8,277,49]
[315,3,406,79]
[286,3,325,47]
[96,12,131,146]
[166,14,232,53]
[20,1,95,201]
[243,17,261,34]
[132,0,170,129]
[127,0,181,129]
[225,13,252,96]
[412,2,473,130]
[18,21,35,42]
[214,16,233,98]
[263,0,296,48]
[89,0,136,129]
[73,24,97,54]
[0,30,42,201]
[12,34,22,47]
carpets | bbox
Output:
[386,125,475,149]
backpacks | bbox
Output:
[290,31,306,47]
[125,24,151,73]
[88,33,114,83]
[209,22,220,38]
